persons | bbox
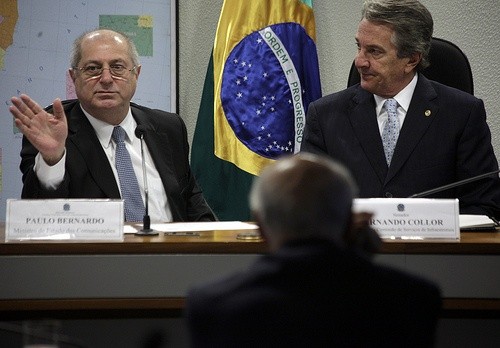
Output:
[185,154,444,348]
[8,29,219,226]
[299,0,500,225]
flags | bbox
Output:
[187,0,323,225]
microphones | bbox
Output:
[134,126,160,236]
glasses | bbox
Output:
[74,63,137,76]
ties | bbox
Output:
[112,126,146,223]
[381,98,400,168]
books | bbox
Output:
[459,215,500,231]
